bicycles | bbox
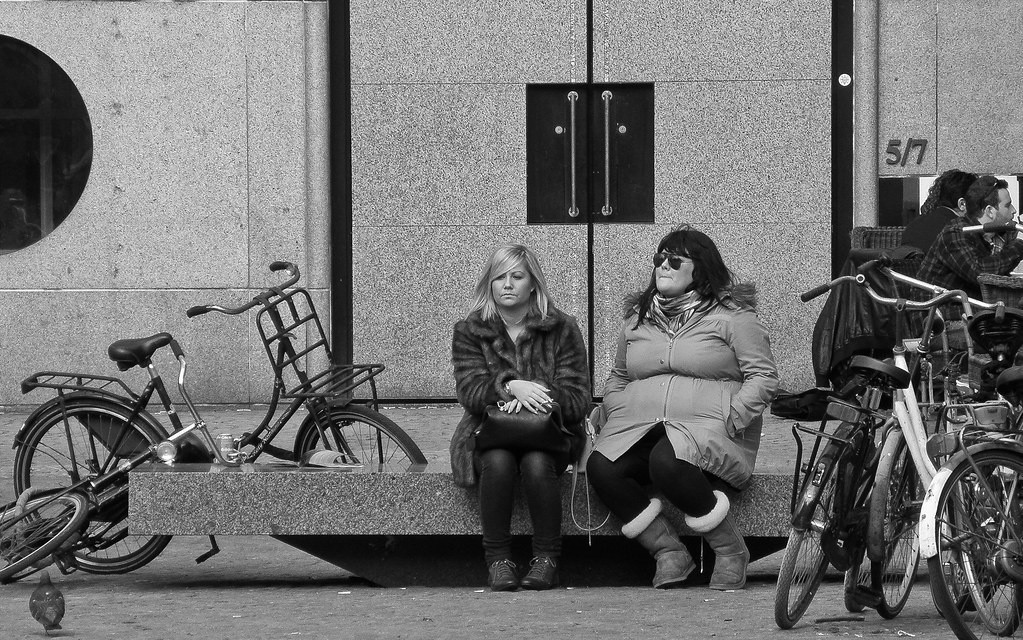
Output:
[11,263,431,577]
[773,213,1023,640]
[0,336,254,583]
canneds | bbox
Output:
[215,433,234,462]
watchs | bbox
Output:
[502,380,511,396]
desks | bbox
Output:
[977,271,1023,334]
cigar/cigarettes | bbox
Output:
[542,399,549,405]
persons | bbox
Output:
[585,220,780,591]
[900,168,980,254]
[449,244,592,591]
[909,176,1023,401]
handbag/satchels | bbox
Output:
[771,390,840,421]
[474,406,575,455]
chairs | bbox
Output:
[851,225,909,251]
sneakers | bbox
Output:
[488,559,518,591]
[520,556,557,590]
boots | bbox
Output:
[621,497,696,589]
[685,490,750,589]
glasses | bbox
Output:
[653,253,694,270]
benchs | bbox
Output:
[128,463,917,587]
